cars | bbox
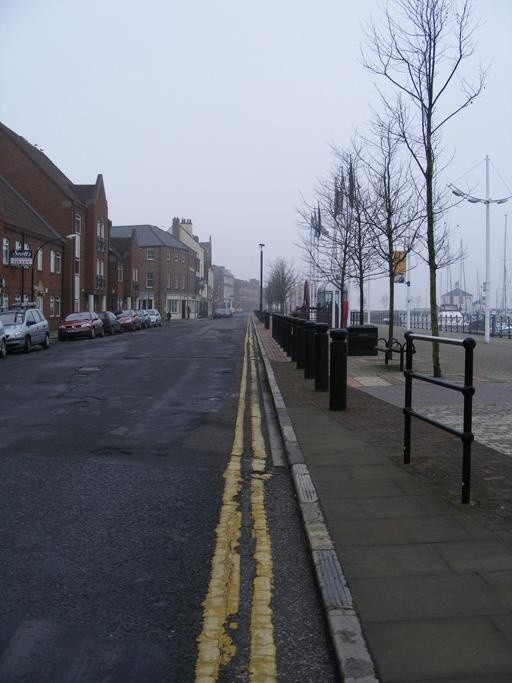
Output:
[212,307,236,320]
[58,309,161,341]
[0,309,53,358]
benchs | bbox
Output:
[374,338,416,372]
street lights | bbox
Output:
[259,243,265,311]
[29,230,77,307]
